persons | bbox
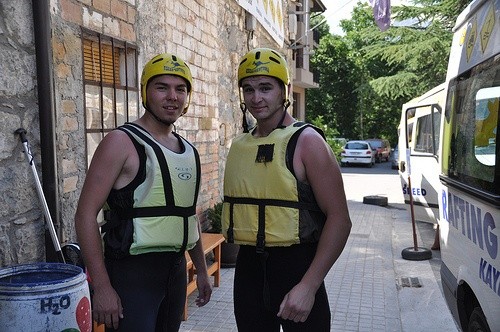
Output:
[220,48,352,332]
[75,53,212,332]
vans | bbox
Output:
[397,80,500,225]
[437,0,500,332]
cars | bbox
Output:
[340,140,376,168]
[391,144,399,170]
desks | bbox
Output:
[184,233,224,321]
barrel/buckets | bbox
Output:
[0,262,92,332]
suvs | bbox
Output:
[364,138,391,163]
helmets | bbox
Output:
[238,48,289,86]
[141,53,193,109]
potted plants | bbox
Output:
[200,196,238,266]
